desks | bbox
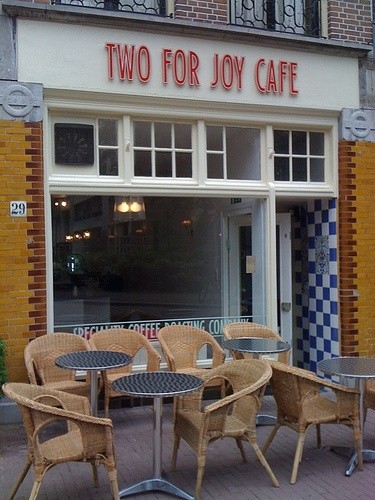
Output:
[223,337,290,356]
[56,349,133,416]
[110,373,204,500]
[316,358,375,476]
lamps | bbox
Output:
[114,195,145,223]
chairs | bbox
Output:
[0,323,375,500]
